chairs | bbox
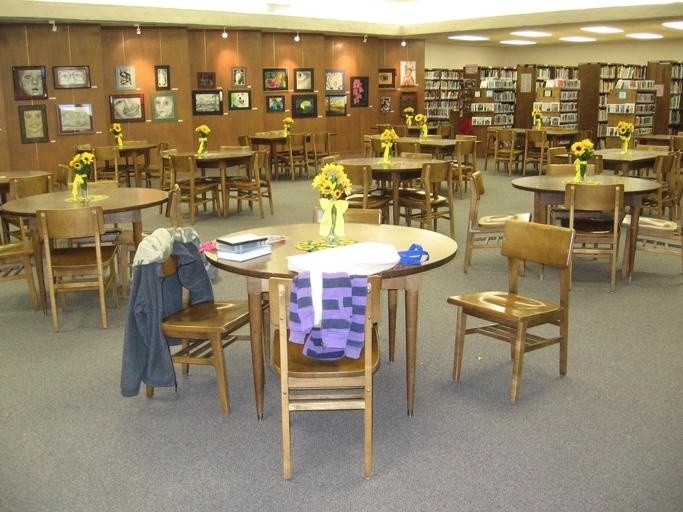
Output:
[120,227,269,414]
[269,275,381,480]
[448,221,576,406]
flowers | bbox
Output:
[415,114,428,139]
[531,109,542,128]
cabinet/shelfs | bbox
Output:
[607,90,655,147]
[515,65,579,150]
[578,63,654,150]
[425,68,463,122]
[648,62,682,136]
[463,65,517,127]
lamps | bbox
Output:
[48,20,57,31]
[134,23,140,34]
[362,35,369,43]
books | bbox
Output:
[424,63,682,154]
[215,233,272,262]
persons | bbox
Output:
[115,98,141,118]
[402,68,414,86]
[19,70,43,97]
[155,97,173,119]
[25,112,43,137]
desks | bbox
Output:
[202,223,457,420]
